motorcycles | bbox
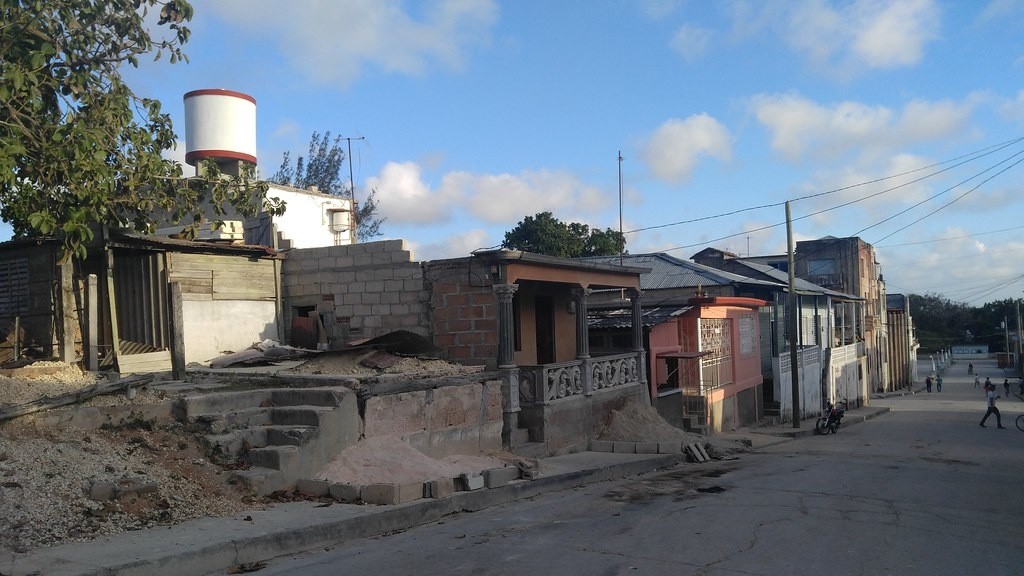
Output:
[816,397,846,435]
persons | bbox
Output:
[968,363,973,375]
[1018,377,1024,393]
[926,377,932,393]
[979,384,1006,429]
[1004,379,1010,398]
[935,375,942,392]
[984,377,991,397]
[974,373,980,388]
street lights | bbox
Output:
[1001,319,1010,367]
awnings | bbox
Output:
[657,350,714,387]
